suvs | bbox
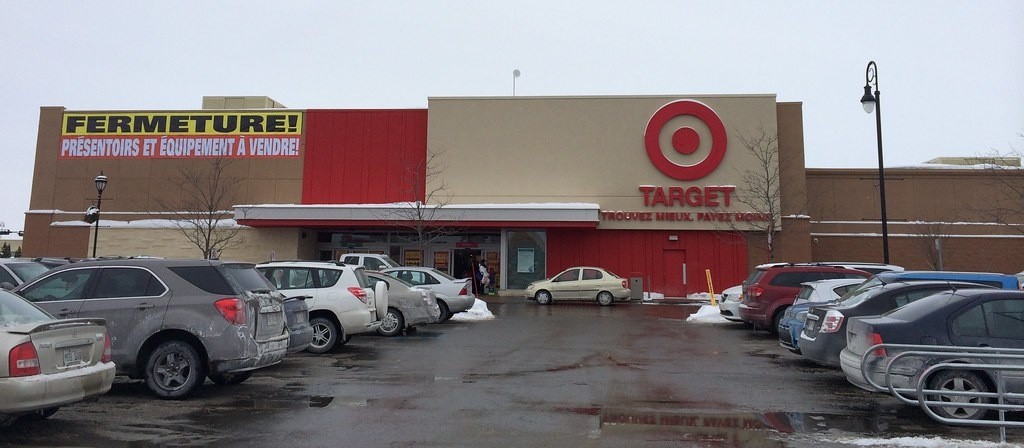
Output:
[717,261,905,323]
[253,260,390,355]
[738,262,899,336]
[7,256,291,399]
[777,269,1022,355]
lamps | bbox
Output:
[669,235,678,241]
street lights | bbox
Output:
[860,60,890,263]
[82,169,108,258]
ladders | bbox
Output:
[465,255,478,299]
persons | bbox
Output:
[478,258,497,296]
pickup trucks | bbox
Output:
[317,252,433,286]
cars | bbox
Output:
[0,254,166,301]
[371,266,476,325]
[524,266,631,307]
[777,272,1022,356]
[798,280,1024,371]
[281,292,314,358]
[839,288,1024,426]
[0,287,117,421]
[297,269,441,337]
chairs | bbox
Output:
[325,272,336,287]
[999,315,1022,339]
[402,273,411,282]
[95,273,115,298]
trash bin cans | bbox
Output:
[630,277,644,300]
